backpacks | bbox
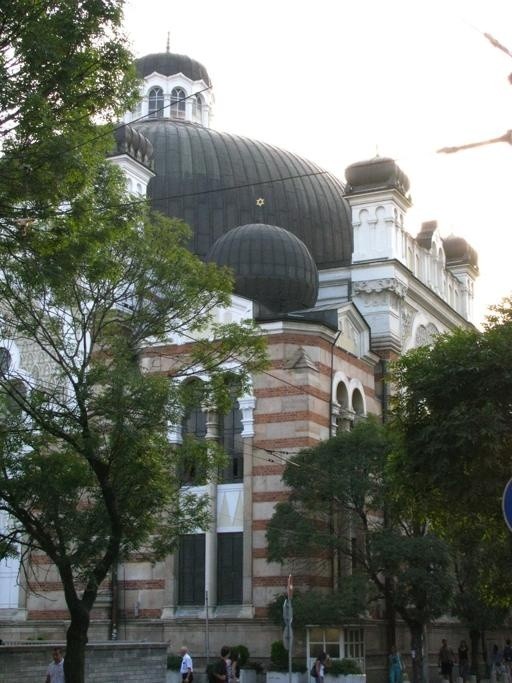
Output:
[207,663,217,683]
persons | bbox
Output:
[228,651,241,682]
[439,637,457,683]
[490,639,512,683]
[410,648,423,683]
[211,645,231,683]
[314,653,326,683]
[458,640,469,677]
[45,646,65,683]
[180,646,194,682]
[387,644,406,683]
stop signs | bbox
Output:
[288,573,294,600]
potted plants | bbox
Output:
[266,640,308,683]
[166,655,184,683]
[324,658,367,683]
[233,645,263,683]
